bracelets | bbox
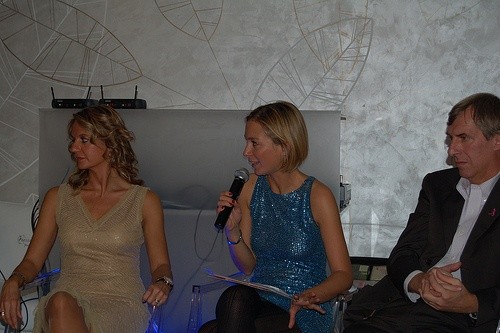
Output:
[227,230,242,245]
[9,272,24,290]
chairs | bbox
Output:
[5,267,157,333]
[187,272,358,333]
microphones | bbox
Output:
[214,168,250,230]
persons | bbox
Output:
[196,99,353,333]
[0,107,174,333]
[343,93,500,333]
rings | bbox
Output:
[153,298,159,303]
[0,311,5,316]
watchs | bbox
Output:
[154,276,173,292]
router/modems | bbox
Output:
[51,86,98,109]
[99,85,146,109]
